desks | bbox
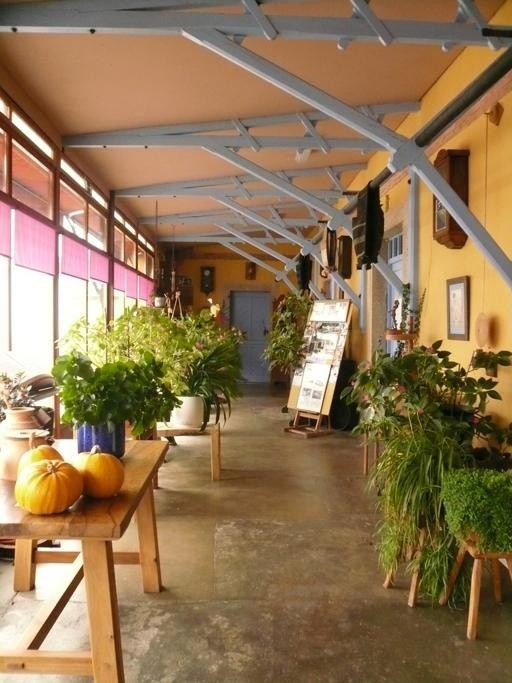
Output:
[1,435,171,682]
[124,419,159,490]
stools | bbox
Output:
[370,482,436,601]
[436,536,512,636]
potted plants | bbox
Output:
[362,404,478,614]
[0,372,50,482]
[55,350,183,464]
[440,468,512,551]
[157,334,241,428]
[261,290,313,397]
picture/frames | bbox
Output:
[445,275,468,341]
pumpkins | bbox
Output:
[16,442,63,479]
[73,444,124,499]
[14,459,85,515]
